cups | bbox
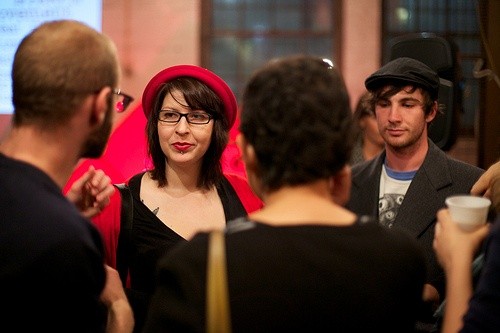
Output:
[445,195,491,233]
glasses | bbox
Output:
[152,110,214,124]
[112,88,134,113]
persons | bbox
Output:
[343,57,488,303]
[433,158,500,333]
[0,20,135,333]
[348,91,386,166]
[89,64,264,333]
[136,55,427,333]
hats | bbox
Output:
[142,64,238,130]
[365,57,440,100]
